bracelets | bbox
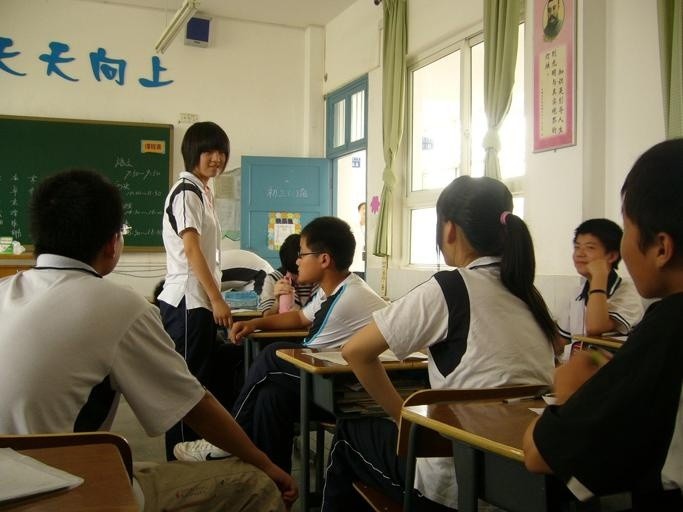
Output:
[587,289,607,296]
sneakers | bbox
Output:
[174,439,237,463]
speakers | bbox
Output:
[181,13,213,49]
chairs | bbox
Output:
[353,385,549,512]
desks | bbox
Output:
[236,326,318,478]
[276,348,427,512]
[400,395,553,511]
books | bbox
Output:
[337,371,429,416]
[0,447,83,510]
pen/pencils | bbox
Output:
[503,392,554,403]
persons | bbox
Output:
[173,215,390,509]
[157,121,233,464]
[542,0,560,38]
[0,170,300,511]
[349,202,367,281]
[523,136,683,511]
[319,175,555,512]
[153,250,277,409]
[548,217,645,369]
[260,232,320,318]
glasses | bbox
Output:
[116,223,132,235]
[297,252,317,256]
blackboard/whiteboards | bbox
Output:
[1,113,173,260]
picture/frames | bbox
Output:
[533,0,579,155]
